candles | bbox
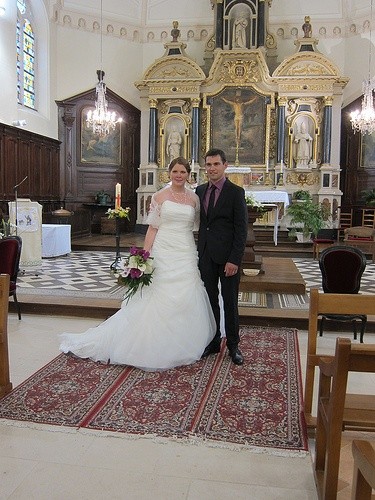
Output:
[281,159,283,172]
[116,182,121,210]
[192,159,195,172]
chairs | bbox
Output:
[319,247,369,343]
[0,234,23,321]
[345,208,375,241]
[337,209,355,241]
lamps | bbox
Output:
[12,119,28,128]
[86,1,123,136]
[349,0,375,132]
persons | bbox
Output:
[101,156,218,369]
[292,121,314,169]
[189,148,247,364]
[166,125,182,166]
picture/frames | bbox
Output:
[203,86,269,167]
[360,128,375,169]
[79,109,125,165]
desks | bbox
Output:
[243,190,290,231]
[41,224,74,258]
[84,203,124,238]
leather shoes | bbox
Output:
[201,345,220,359]
[229,348,244,365]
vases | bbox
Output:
[244,206,272,245]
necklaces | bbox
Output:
[169,184,187,202]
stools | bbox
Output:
[312,239,337,260]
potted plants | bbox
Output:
[285,203,327,242]
[93,189,111,205]
[292,190,311,208]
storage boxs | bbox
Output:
[102,216,119,233]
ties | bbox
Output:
[207,184,218,218]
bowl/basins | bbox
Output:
[243,269,260,277]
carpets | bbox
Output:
[1,322,308,458]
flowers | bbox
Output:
[111,247,157,300]
[105,207,131,221]
[248,194,263,214]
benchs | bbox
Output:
[299,287,375,500]
